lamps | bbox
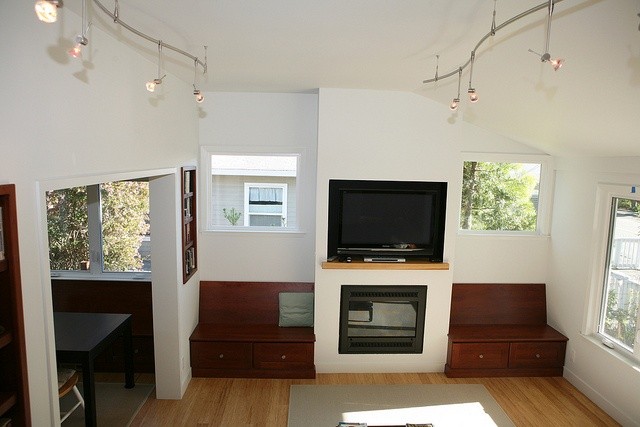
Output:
[66,0,88,60]
[527,1,568,72]
[145,45,168,93]
[34,0,64,24]
[449,69,462,111]
[193,61,205,104]
[466,57,481,104]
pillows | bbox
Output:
[278,292,314,328]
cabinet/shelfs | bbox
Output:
[0,184,33,427]
[445,324,569,379]
[181,165,198,285]
[189,322,316,380]
[99,335,155,372]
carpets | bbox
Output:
[59,380,156,427]
[287,383,516,427]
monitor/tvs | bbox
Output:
[328,179,447,262]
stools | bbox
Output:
[57,368,87,427]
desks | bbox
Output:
[52,309,134,427]
